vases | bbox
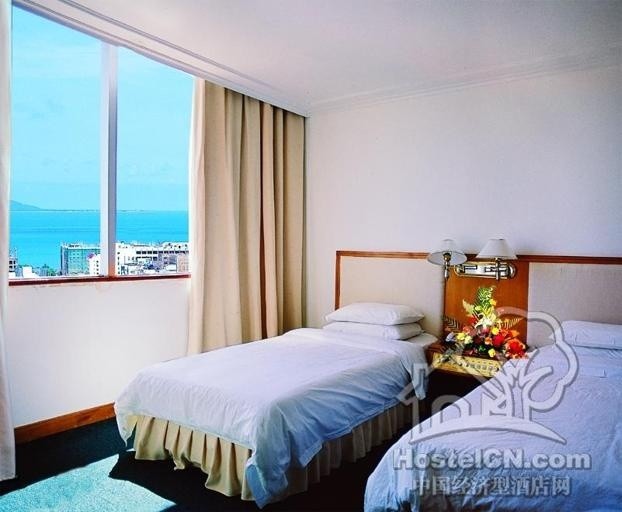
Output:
[467,345,499,355]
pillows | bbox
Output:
[551,345,622,359]
[547,321,622,349]
[324,301,425,326]
[323,322,424,340]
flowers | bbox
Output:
[440,284,527,361]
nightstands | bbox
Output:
[426,340,530,416]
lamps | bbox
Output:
[425,237,517,282]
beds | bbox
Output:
[363,344,622,512]
[112,326,437,512]
